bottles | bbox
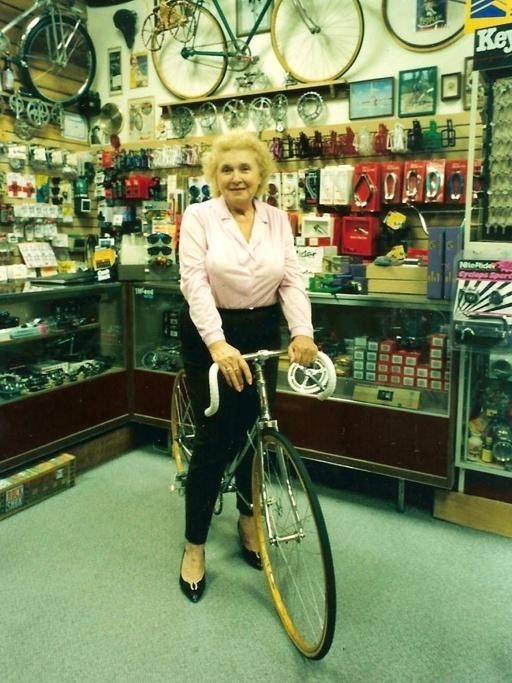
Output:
[353,120,442,155]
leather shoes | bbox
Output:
[179,547,206,604]
[236,519,264,571]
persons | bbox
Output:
[170,125,319,604]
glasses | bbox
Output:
[146,233,172,244]
[151,259,173,270]
[146,246,172,256]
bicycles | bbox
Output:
[0,0,98,107]
[150,0,366,100]
[170,346,337,662]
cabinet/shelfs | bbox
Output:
[130,279,458,513]
[1,279,130,475]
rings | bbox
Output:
[233,367,241,372]
[224,366,232,372]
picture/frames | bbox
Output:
[398,65,438,118]
[106,46,123,96]
[441,72,461,101]
[349,76,394,120]
[127,95,155,143]
[60,109,88,142]
